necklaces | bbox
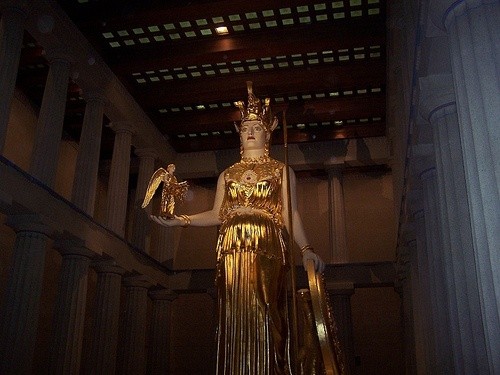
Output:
[240,153,270,163]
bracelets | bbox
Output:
[183,215,192,227]
[301,246,314,255]
[179,214,188,227]
[299,244,311,254]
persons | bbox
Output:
[150,117,328,375]
[158,163,180,221]
